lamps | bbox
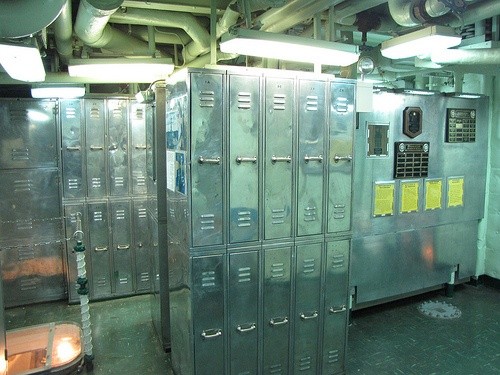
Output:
[0,41,46,81]
[219,28,360,67]
[68,58,174,83]
[380,25,463,60]
[31,84,85,99]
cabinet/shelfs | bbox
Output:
[61,67,357,375]
